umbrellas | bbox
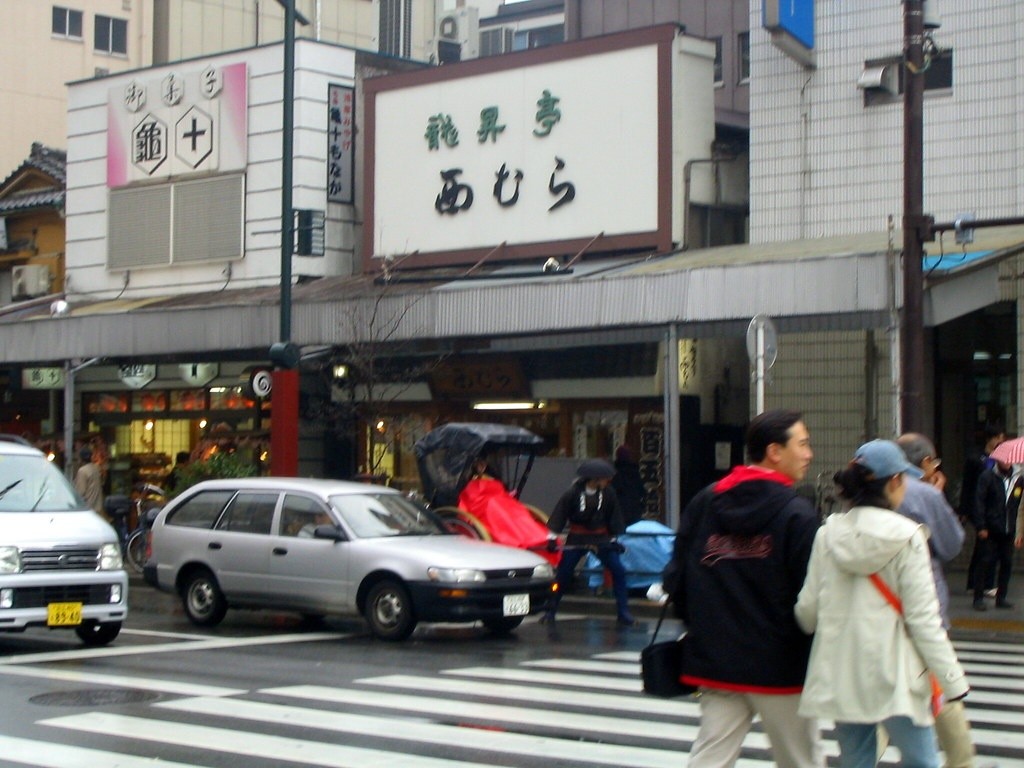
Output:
[989,436,1024,465]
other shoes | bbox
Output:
[617,613,637,624]
[973,600,988,611]
[985,587,998,598]
[996,600,1015,608]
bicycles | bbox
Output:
[103,482,165,574]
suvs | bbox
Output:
[0,431,131,649]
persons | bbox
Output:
[794,439,969,768]
[75,449,103,515]
[959,429,1024,610]
[297,502,332,538]
[162,452,193,493]
[457,447,490,486]
[537,459,637,625]
[875,434,1003,768]
[662,408,830,768]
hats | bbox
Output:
[577,457,616,480]
[854,438,923,482]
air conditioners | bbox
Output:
[11,264,50,299]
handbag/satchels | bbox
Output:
[641,597,699,697]
[929,672,945,716]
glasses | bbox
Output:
[926,455,942,469]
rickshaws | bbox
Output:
[412,420,628,572]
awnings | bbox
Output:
[0,224,1024,364]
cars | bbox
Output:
[144,475,558,643]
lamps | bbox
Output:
[50,269,131,321]
[543,231,603,271]
